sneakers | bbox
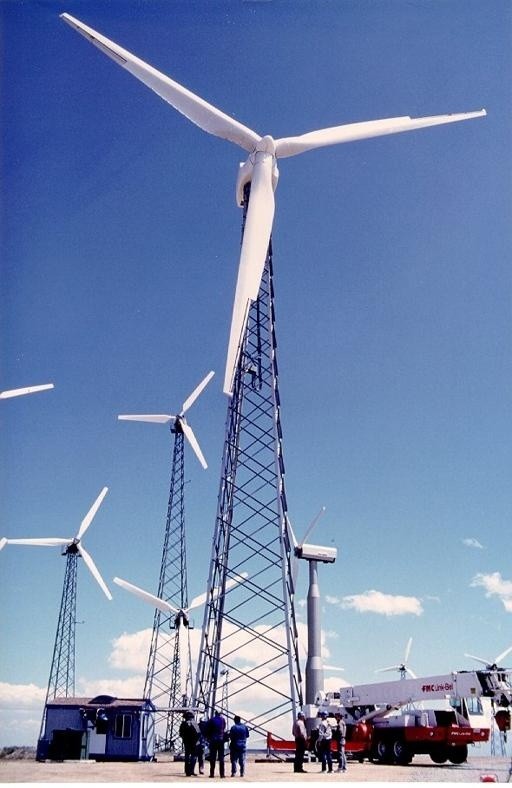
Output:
[320,769,348,773]
[209,775,243,778]
[294,769,308,773]
[187,772,204,776]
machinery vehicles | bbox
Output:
[265,667,512,767]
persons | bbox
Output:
[334,713,347,773]
[179,712,209,776]
[229,716,249,776]
[319,711,334,773]
[292,712,308,773]
[206,711,226,777]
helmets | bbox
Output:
[321,711,329,716]
[299,712,305,717]
